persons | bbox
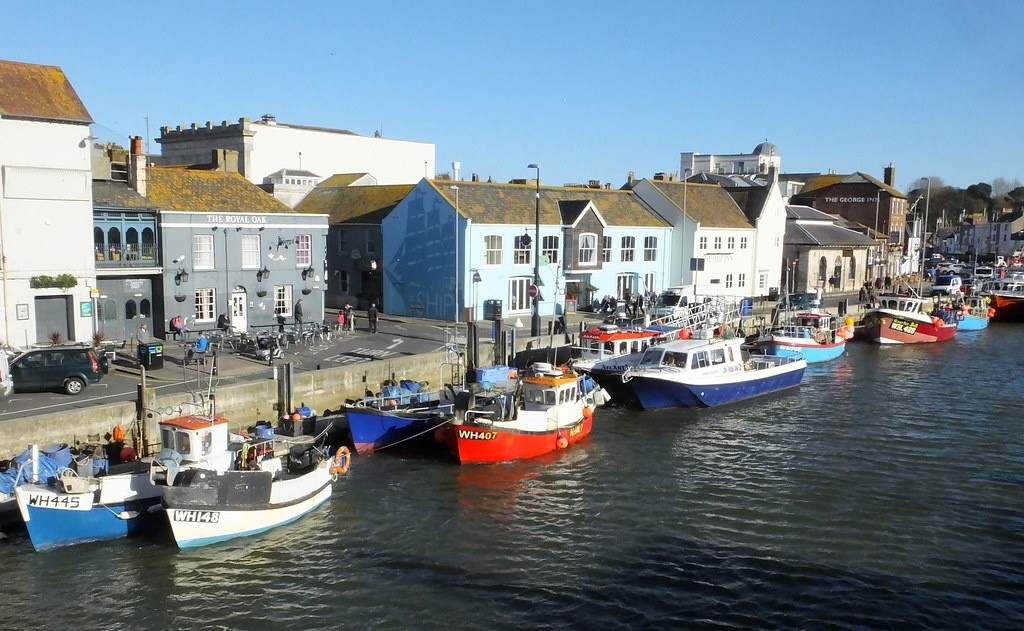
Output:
[867,286,874,303]
[885,274,891,290]
[336,303,354,331]
[601,293,617,314]
[924,266,936,282]
[829,275,834,293]
[859,287,866,302]
[171,314,185,342]
[294,299,305,332]
[368,303,379,335]
[624,291,657,315]
[876,275,881,289]
[218,313,236,335]
[187,331,208,358]
[278,314,286,332]
[137,322,150,343]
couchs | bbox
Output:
[97,253,104,261]
[112,252,120,260]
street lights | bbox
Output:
[679,167,695,285]
[919,177,931,298]
[527,163,541,337]
[449,184,459,350]
[874,188,887,241]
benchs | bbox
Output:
[165,327,224,340]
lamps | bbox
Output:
[277,236,299,251]
[307,266,314,277]
[179,268,189,282]
[84,134,98,140]
[260,266,270,279]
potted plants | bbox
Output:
[93,329,105,350]
[30,274,76,288]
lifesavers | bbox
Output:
[813,329,823,343]
[333,445,350,474]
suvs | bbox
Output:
[9,344,109,395]
[958,265,973,277]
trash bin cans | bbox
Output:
[487,299,502,321]
[769,286,778,301]
[137,342,163,371]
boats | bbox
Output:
[973,271,1024,319]
[561,298,742,388]
[149,438,352,550]
[605,327,807,411]
[753,301,855,363]
[931,287,997,330]
[11,409,237,552]
[342,360,474,456]
[859,273,960,344]
[430,361,612,466]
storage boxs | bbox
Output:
[476,364,508,382]
[417,392,439,402]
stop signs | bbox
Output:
[528,283,539,297]
[473,273,480,282]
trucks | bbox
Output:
[649,291,689,325]
[974,265,994,282]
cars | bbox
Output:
[947,263,967,275]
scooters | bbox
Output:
[254,335,285,362]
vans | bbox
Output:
[929,275,962,296]
[937,263,953,274]
[0,347,14,402]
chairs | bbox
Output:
[194,323,339,364]
